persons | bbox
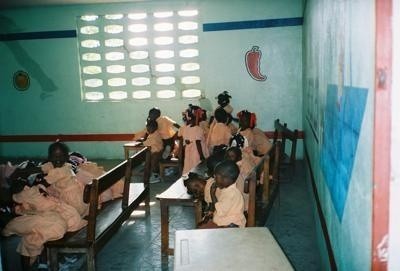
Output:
[133,90,273,229]
[0,142,124,271]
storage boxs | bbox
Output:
[173,227,296,271]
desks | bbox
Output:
[155,155,212,256]
[123,141,145,160]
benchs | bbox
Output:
[44,146,152,271]
[244,141,282,227]
[159,136,183,183]
[273,119,298,184]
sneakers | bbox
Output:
[33,254,48,269]
[58,254,79,271]
[162,145,171,159]
[149,167,176,184]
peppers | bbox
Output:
[244,44,267,82]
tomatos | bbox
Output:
[12,70,31,91]
